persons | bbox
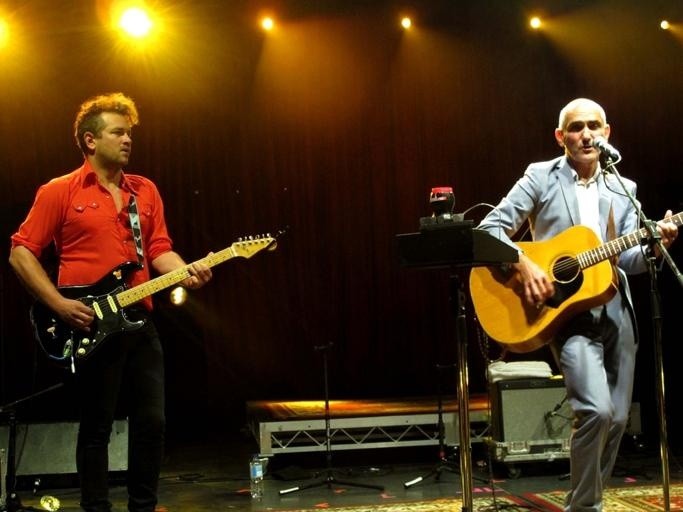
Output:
[471,97,680,512]
[7,93,211,512]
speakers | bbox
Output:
[15,417,130,489]
[486,379,576,442]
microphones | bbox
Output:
[545,400,563,421]
[593,134,621,158]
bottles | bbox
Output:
[249,453,264,498]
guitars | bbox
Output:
[469,210,683,354]
[29,232,278,360]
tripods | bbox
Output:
[402,362,492,492]
[276,343,385,496]
